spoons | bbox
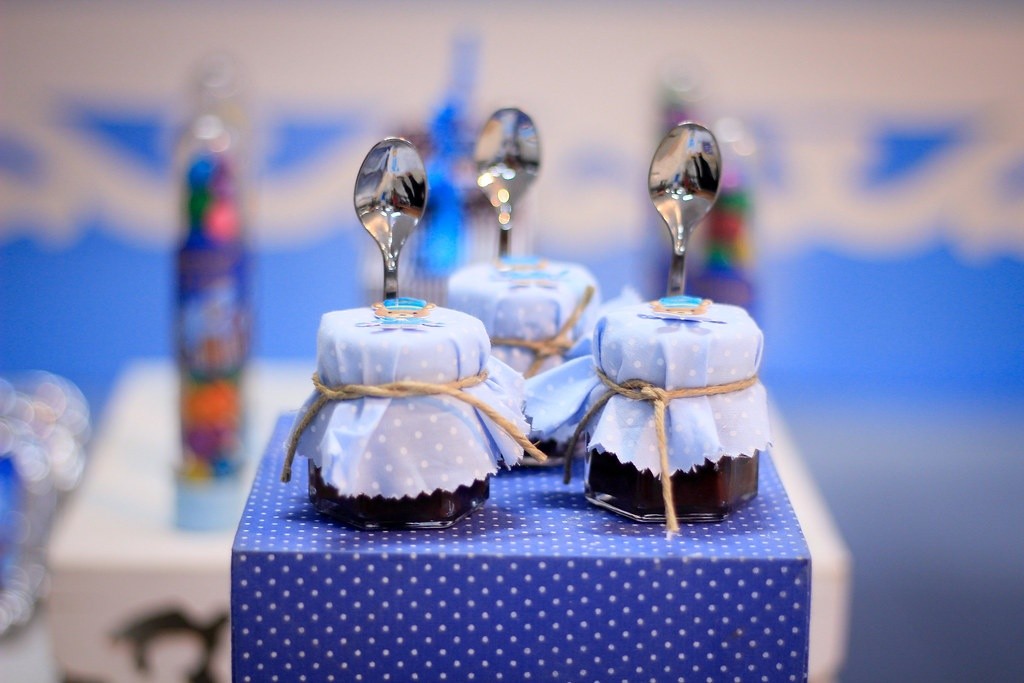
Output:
[648,120,723,295]
[353,136,428,302]
[471,106,541,257]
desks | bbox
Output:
[47,365,849,683]
